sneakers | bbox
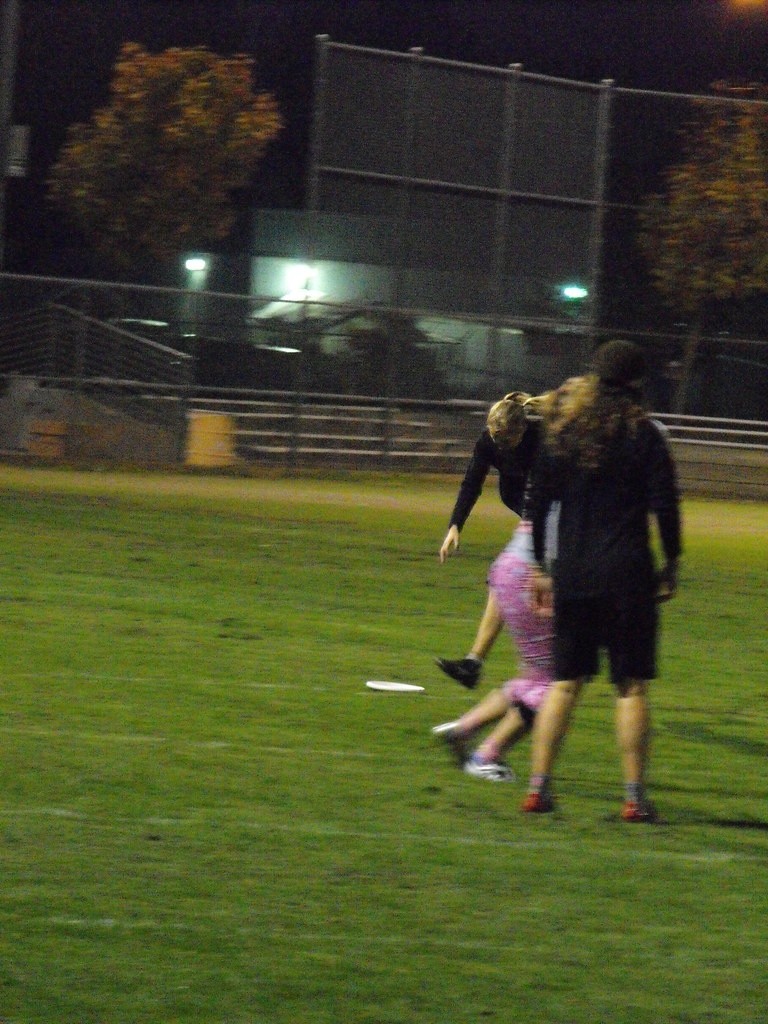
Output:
[522,788,550,812]
[435,657,481,689]
[464,758,516,783]
[432,721,469,764]
[624,801,666,824]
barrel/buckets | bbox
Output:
[183,409,239,466]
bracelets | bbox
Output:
[532,564,546,569]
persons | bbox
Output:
[432,377,597,783]
[523,341,682,822]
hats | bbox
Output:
[594,339,651,395]
